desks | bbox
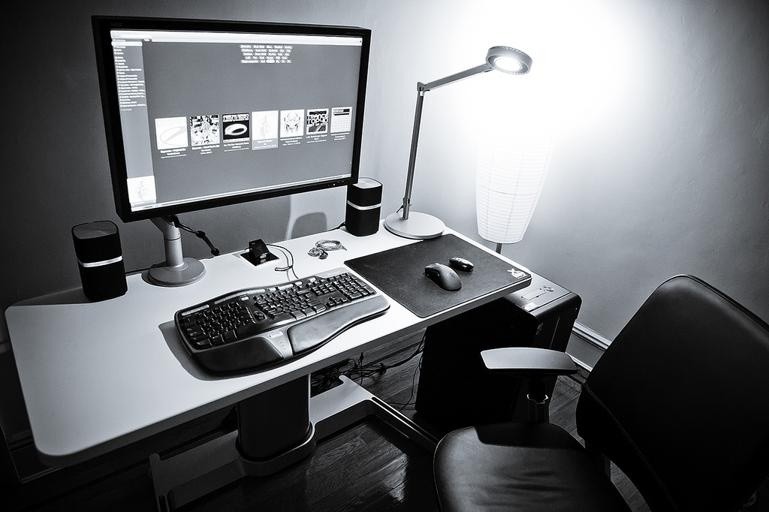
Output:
[4,219,531,512]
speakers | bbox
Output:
[345,177,382,237]
[72,219,128,303]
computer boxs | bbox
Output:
[413,265,583,436]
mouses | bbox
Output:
[425,263,462,292]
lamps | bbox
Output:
[384,46,532,240]
[475,134,549,253]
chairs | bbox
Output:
[432,274,768,512]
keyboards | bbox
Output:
[174,266,390,376]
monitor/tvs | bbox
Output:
[89,13,372,224]
